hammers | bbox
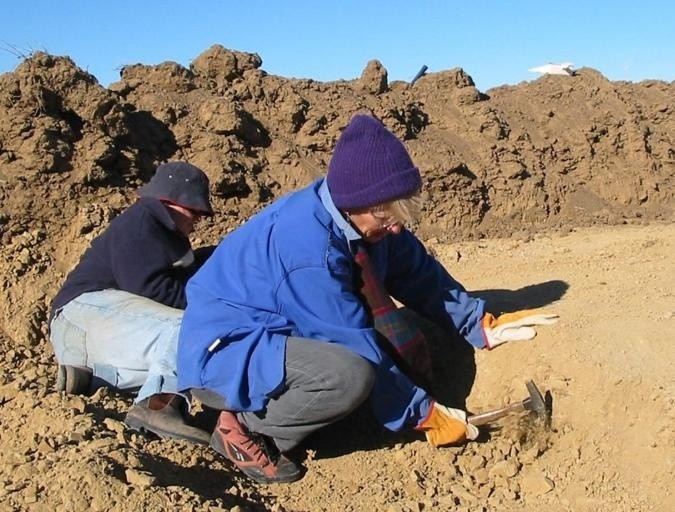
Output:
[468,380,548,429]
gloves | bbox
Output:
[413,399,479,447]
[481,307,560,351]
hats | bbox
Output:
[134,161,214,216]
[327,114,423,209]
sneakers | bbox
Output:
[57,364,91,393]
[124,393,301,483]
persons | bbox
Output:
[175,113,564,484]
[50,162,212,447]
